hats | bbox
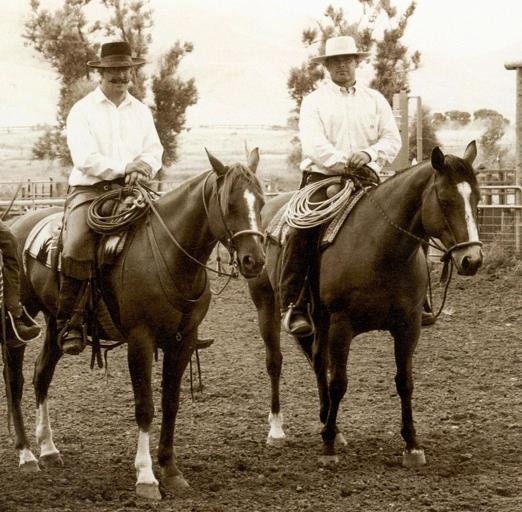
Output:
[86,41,147,69]
[310,36,372,63]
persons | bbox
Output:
[1,220,41,351]
[54,43,214,353]
[278,36,435,334]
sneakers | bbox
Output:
[422,309,436,327]
[6,318,41,346]
[61,337,83,353]
[289,313,312,334]
[196,338,215,348]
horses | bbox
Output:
[245,140,485,471]
[0,146,267,501]
